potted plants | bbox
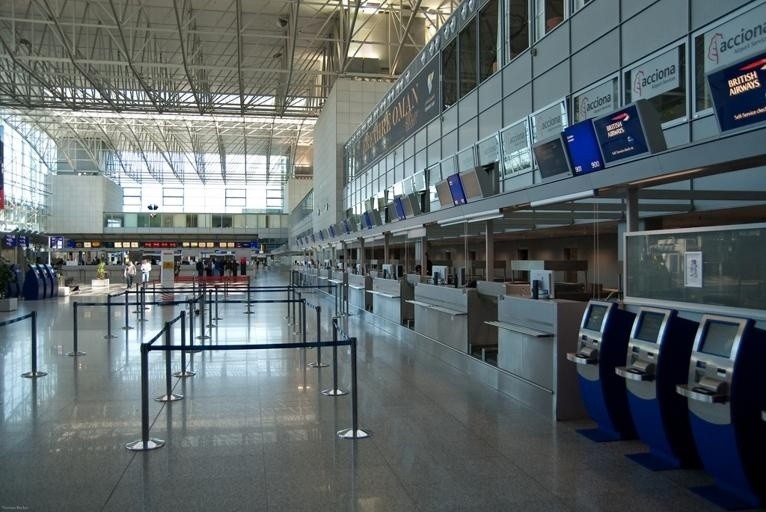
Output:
[90,262,109,286]
[0,255,19,313]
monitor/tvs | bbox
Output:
[294,167,492,246]
[706,49,766,135]
[697,318,740,359]
[633,310,665,343]
[531,99,667,186]
[432,266,449,286]
[295,259,393,279]
[530,269,555,299]
[584,304,608,332]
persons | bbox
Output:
[196,258,239,283]
[140,259,152,282]
[123,261,136,289]
